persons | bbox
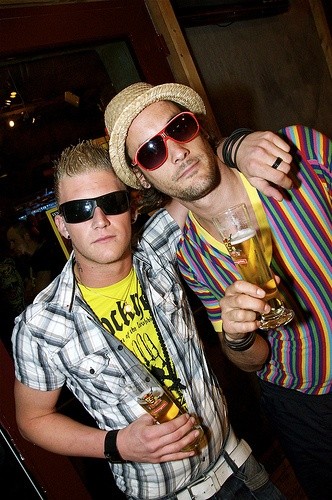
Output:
[6,210,68,301]
[103,82,332,500]
[10,127,293,500]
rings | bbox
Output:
[272,157,283,169]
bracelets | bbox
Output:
[222,331,256,351]
[222,128,256,174]
[104,430,133,465]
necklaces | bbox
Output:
[72,266,134,312]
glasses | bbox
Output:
[59,190,132,224]
[130,112,200,172]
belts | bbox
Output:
[171,425,252,500]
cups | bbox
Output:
[211,203,294,331]
[118,364,204,453]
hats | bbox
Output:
[104,82,206,191]
